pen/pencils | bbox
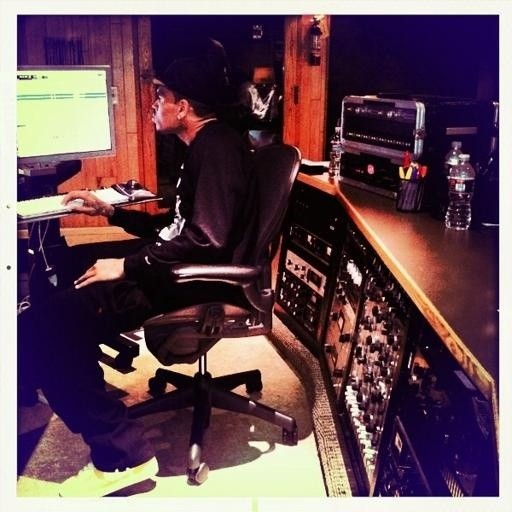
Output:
[398,151,429,212]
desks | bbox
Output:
[277,165,499,497]
[17,183,162,372]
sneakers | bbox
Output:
[59,457,158,497]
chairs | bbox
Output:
[143,141,297,484]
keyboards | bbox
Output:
[16,188,130,220]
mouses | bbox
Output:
[125,179,141,191]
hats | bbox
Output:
[153,36,227,106]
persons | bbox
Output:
[20,39,248,497]
[237,54,284,150]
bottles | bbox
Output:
[442,141,475,232]
[328,127,342,181]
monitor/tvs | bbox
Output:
[17,64,116,175]
